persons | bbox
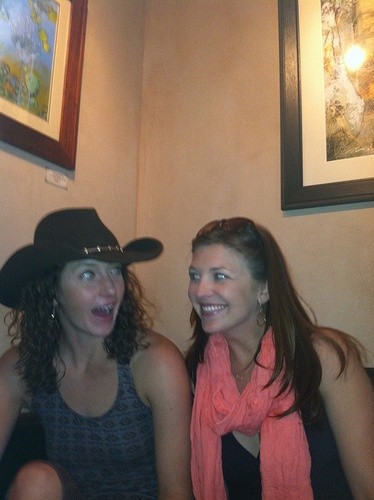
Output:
[183,217,374,500]
[0,206,194,500]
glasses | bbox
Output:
[195,217,268,275]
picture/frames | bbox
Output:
[1,0,88,171]
[277,0,374,212]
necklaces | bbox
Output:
[223,323,262,381]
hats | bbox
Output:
[0,208,163,308]
